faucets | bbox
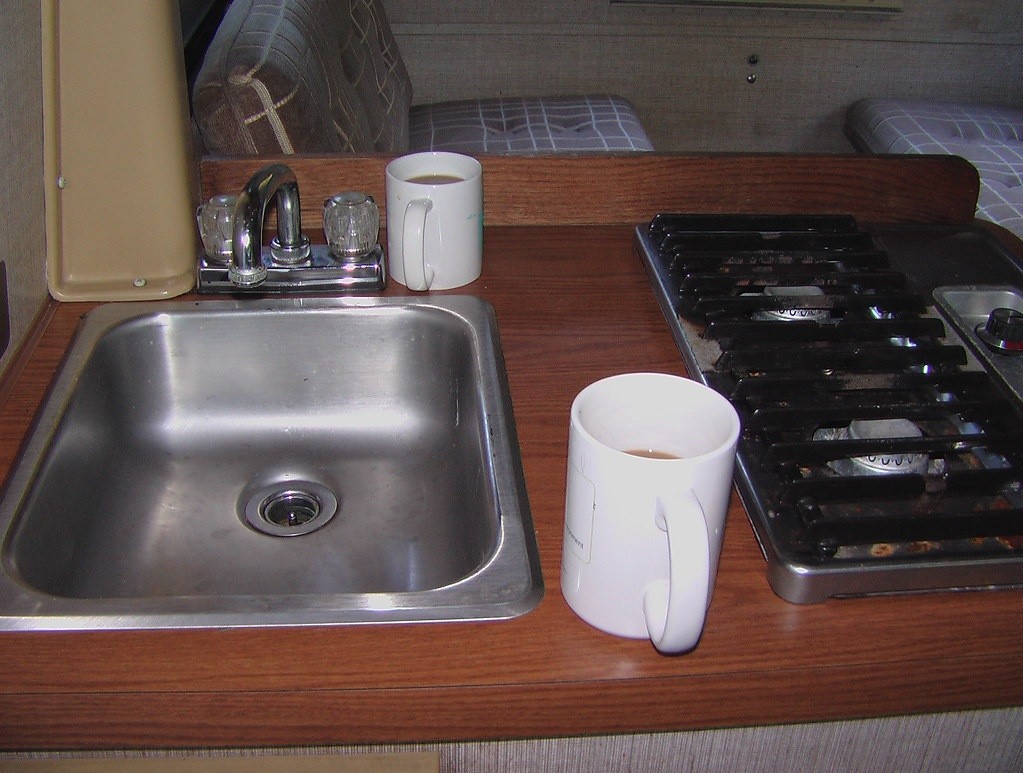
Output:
[223,165,311,289]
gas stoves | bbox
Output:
[631,210,1023,604]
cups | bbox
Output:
[559,371,741,651]
[384,151,484,291]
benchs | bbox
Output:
[189,0,653,153]
[842,93,1023,240]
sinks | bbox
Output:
[0,292,546,635]
